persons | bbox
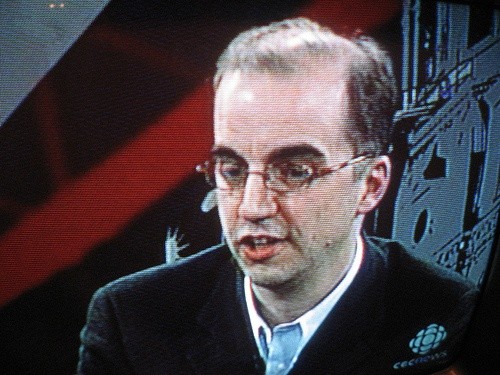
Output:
[76,18,500,375]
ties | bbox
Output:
[259,323,302,375]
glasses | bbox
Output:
[195,152,374,193]
